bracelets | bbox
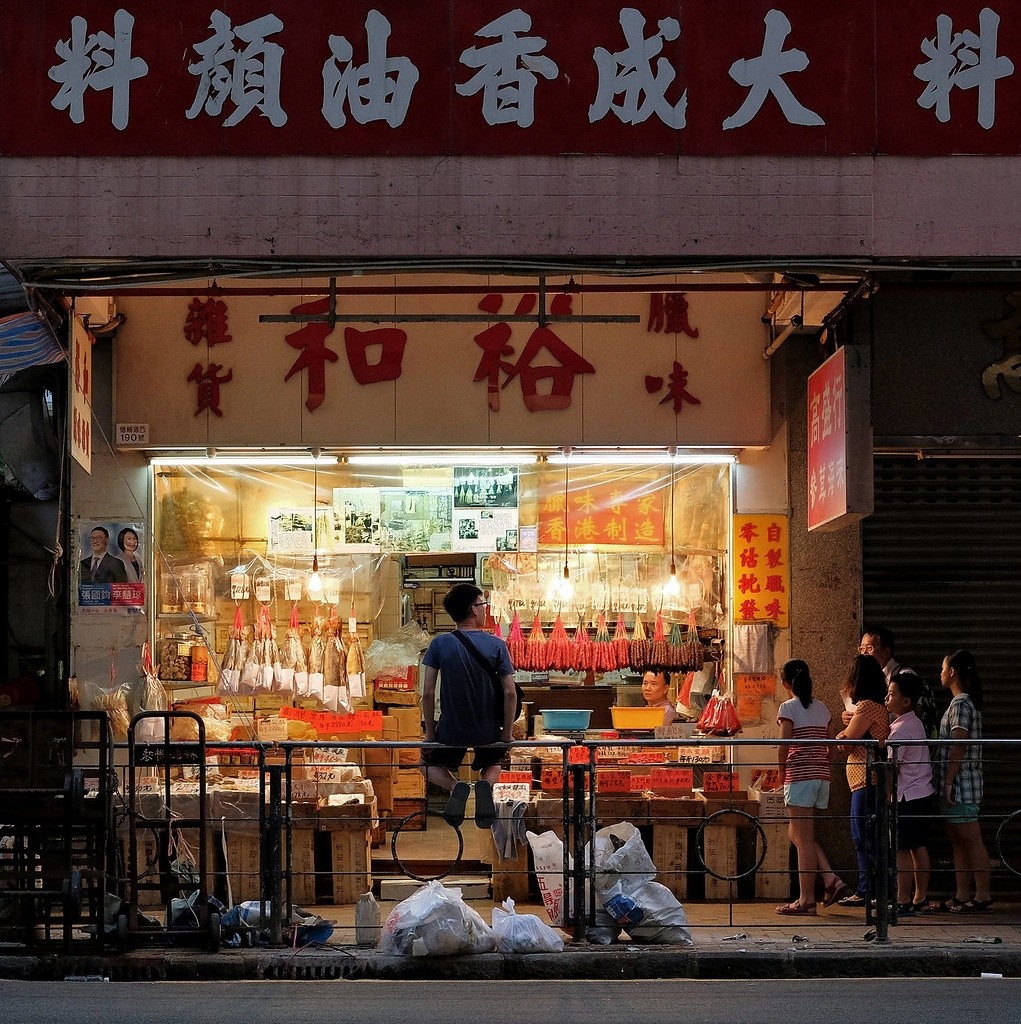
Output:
[946,781,953,785]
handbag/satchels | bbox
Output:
[495,677,524,727]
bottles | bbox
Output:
[160,633,209,681]
[158,573,181,614]
[179,570,207,614]
[354,891,381,945]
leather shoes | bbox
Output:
[838,897,865,907]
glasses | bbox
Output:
[857,645,880,652]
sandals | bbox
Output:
[823,877,847,907]
[777,900,818,916]
[932,898,995,913]
[890,899,931,917]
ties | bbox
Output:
[90,557,100,582]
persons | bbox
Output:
[362,514,372,544]
[78,525,128,583]
[928,650,996,914]
[775,657,848,917]
[833,654,893,909]
[114,527,145,583]
[840,624,924,909]
[417,583,517,829]
[496,531,518,551]
[874,672,937,917]
[642,669,678,727]
[461,519,472,539]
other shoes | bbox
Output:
[444,780,496,829]
